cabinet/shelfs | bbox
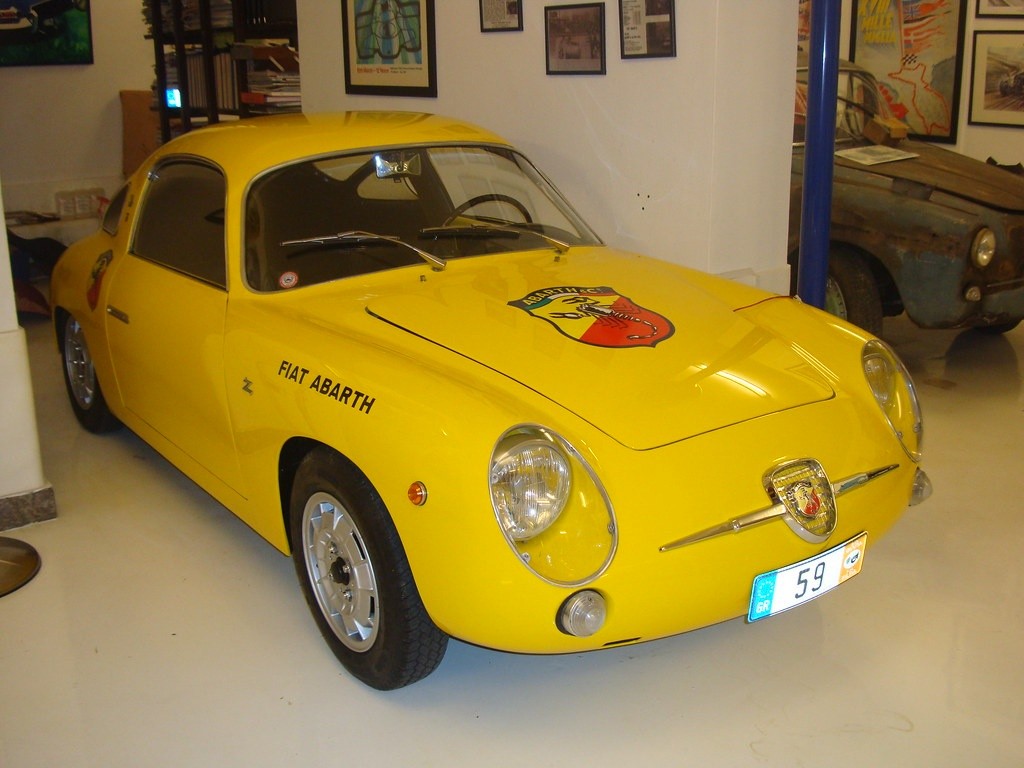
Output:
[142,1,301,149]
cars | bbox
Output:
[52,105,931,690]
[789,53,1023,347]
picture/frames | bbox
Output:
[339,0,439,99]
[848,0,968,147]
[974,0,1024,19]
[480,1,524,33]
[966,29,1024,128]
[618,0,678,60]
[544,2,608,78]
[0,0,95,70]
